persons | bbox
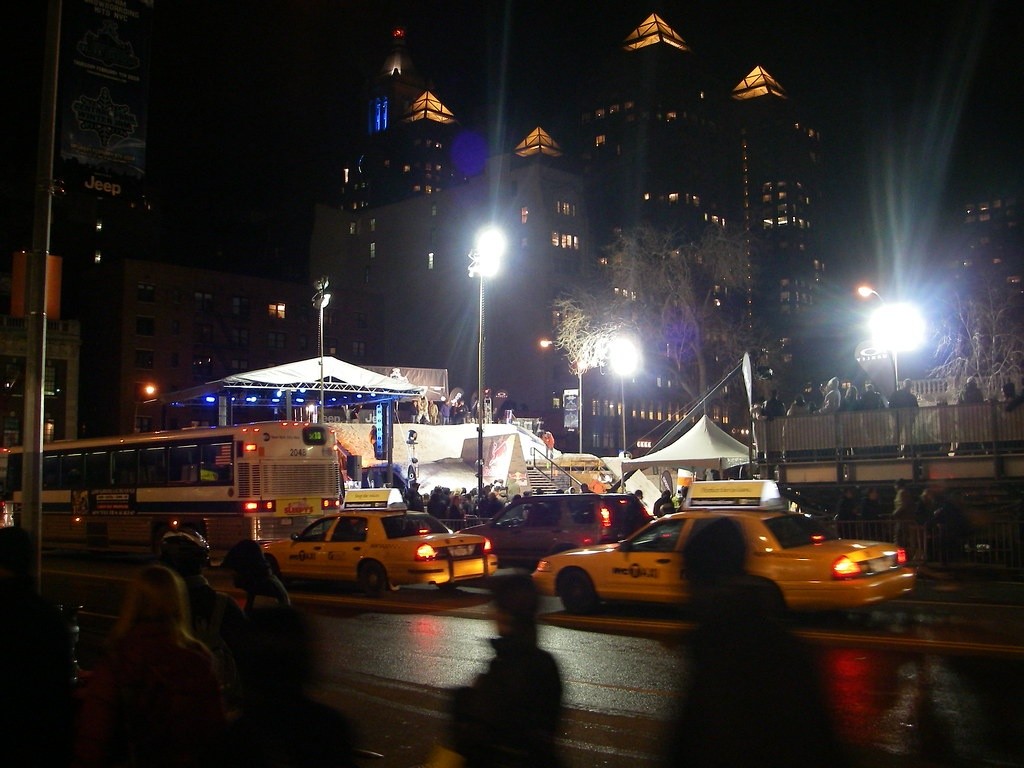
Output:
[653,465,751,518]
[760,376,1024,459]
[542,430,555,459]
[833,477,962,562]
[0,529,355,768]
[665,518,850,768]
[365,458,657,522]
[350,403,362,423]
[445,573,563,768]
[411,393,479,425]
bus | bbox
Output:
[11,421,343,571]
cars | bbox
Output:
[530,512,916,630]
[258,508,498,599]
[455,493,650,569]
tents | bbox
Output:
[621,415,756,494]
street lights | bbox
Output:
[611,351,637,457]
[314,295,330,422]
[857,287,902,453]
[473,226,507,498]
[541,340,582,453]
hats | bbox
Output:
[828,376,838,390]
[223,538,266,575]
[684,516,745,573]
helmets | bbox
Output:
[151,527,210,570]
